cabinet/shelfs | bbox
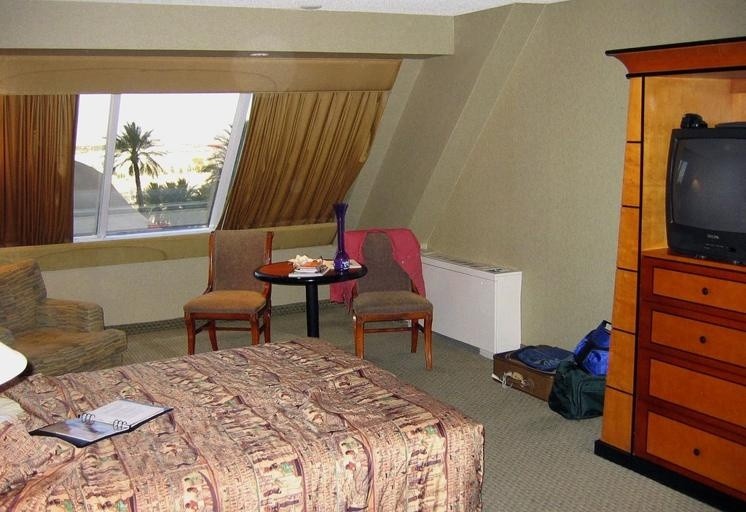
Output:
[593,36,745,512]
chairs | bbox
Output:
[184,229,275,355]
[342,227,435,369]
[0,257,128,377]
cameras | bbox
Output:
[681,113,707,128]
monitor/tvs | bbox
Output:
[666,128,746,261]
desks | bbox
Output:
[253,258,368,337]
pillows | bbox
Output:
[0,387,82,499]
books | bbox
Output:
[27,398,174,448]
[288,258,362,276]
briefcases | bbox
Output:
[493,345,574,402]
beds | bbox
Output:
[0,336,486,512]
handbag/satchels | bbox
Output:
[549,321,610,420]
